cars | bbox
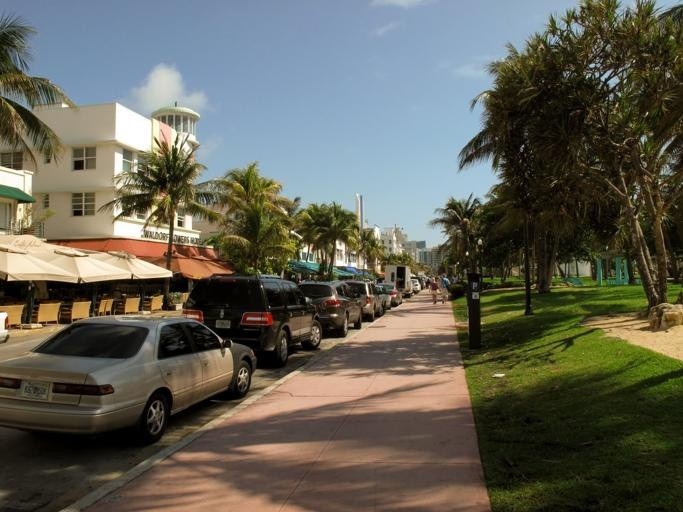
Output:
[411,276,426,294]
[1,314,257,444]
[298,279,403,338]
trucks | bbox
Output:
[384,265,412,297]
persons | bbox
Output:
[426,273,459,305]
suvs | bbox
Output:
[185,273,322,368]
